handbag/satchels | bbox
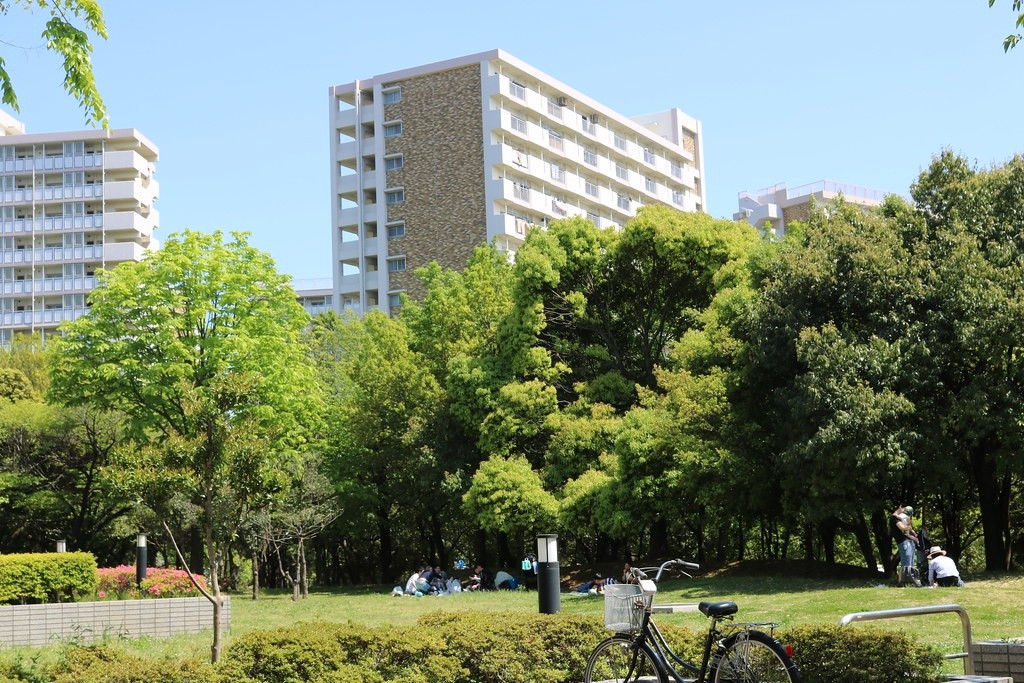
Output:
[522,558,532,570]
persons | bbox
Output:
[406,565,494,594]
[570,562,646,593]
[888,498,965,588]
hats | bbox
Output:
[927,545,946,558]
[904,505,914,514]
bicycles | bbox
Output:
[580,560,801,683]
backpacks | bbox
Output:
[899,565,920,587]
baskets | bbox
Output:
[603,583,650,632]
